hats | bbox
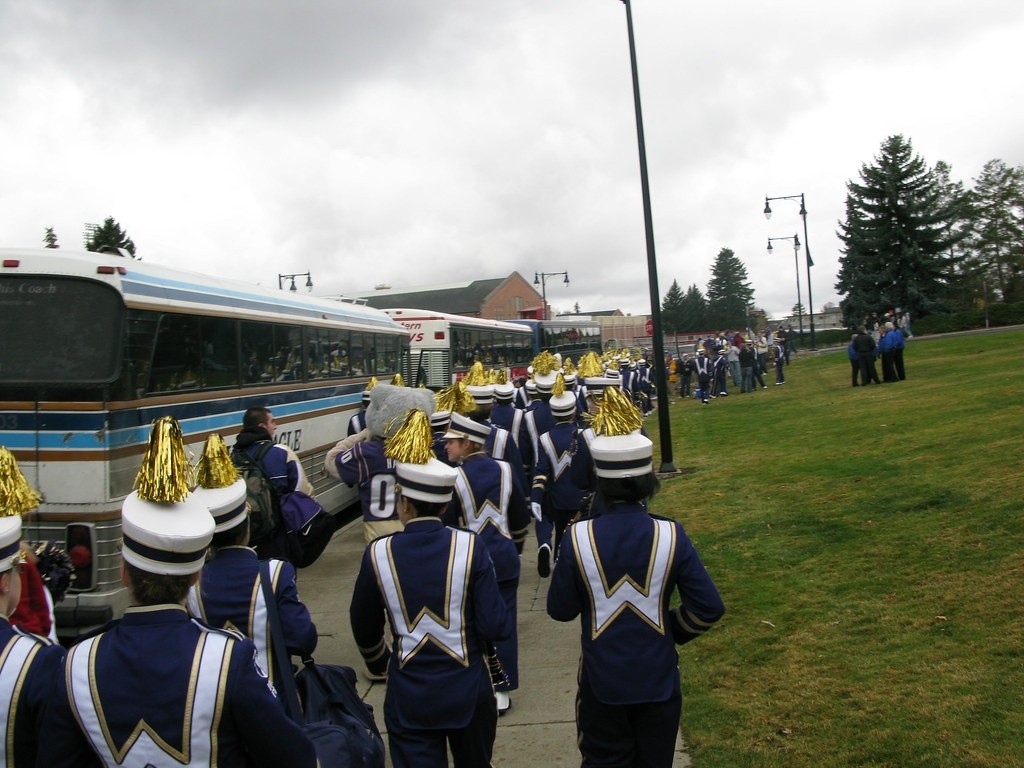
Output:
[0,447,47,571]
[362,346,654,479]
[185,431,251,535]
[385,409,458,503]
[122,413,217,577]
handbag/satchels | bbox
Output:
[273,491,336,569]
[298,662,388,768]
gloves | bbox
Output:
[530,502,542,522]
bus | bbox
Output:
[380,308,532,393]
[506,321,602,368]
[0,247,410,648]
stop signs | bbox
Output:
[646,320,653,336]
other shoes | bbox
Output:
[537,544,552,579]
[495,691,512,717]
[644,411,648,417]
[670,379,789,404]
[650,396,658,400]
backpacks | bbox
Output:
[237,440,278,543]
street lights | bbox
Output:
[534,271,570,319]
[767,233,804,349]
[279,271,313,294]
[764,194,818,352]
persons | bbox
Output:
[39,483,319,768]
[325,347,679,714]
[185,476,318,699]
[679,326,798,405]
[228,407,315,563]
[350,455,514,768]
[0,509,91,768]
[151,342,527,390]
[848,309,914,387]
[547,430,725,768]
